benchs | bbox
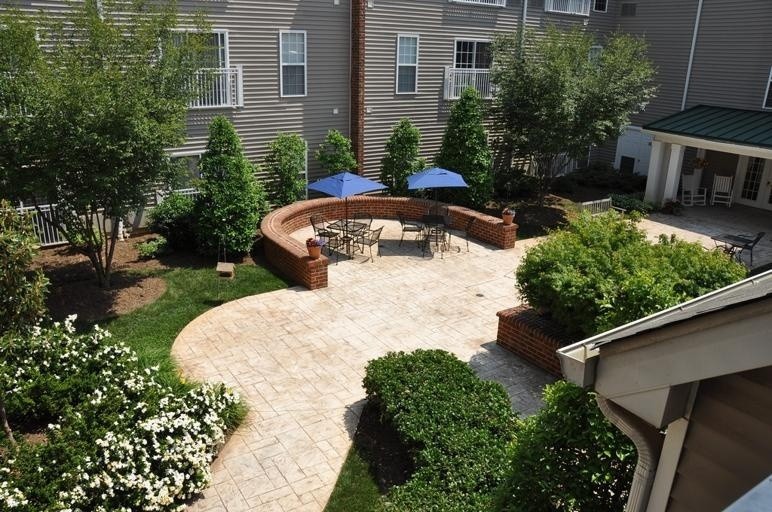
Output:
[580,196,628,217]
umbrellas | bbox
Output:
[307,172,389,254]
[406,167,471,248]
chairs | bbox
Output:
[679,171,707,207]
[736,232,767,266]
[710,172,735,208]
[309,201,477,266]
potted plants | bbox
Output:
[502,207,515,225]
[306,237,323,260]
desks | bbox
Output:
[711,233,752,258]
